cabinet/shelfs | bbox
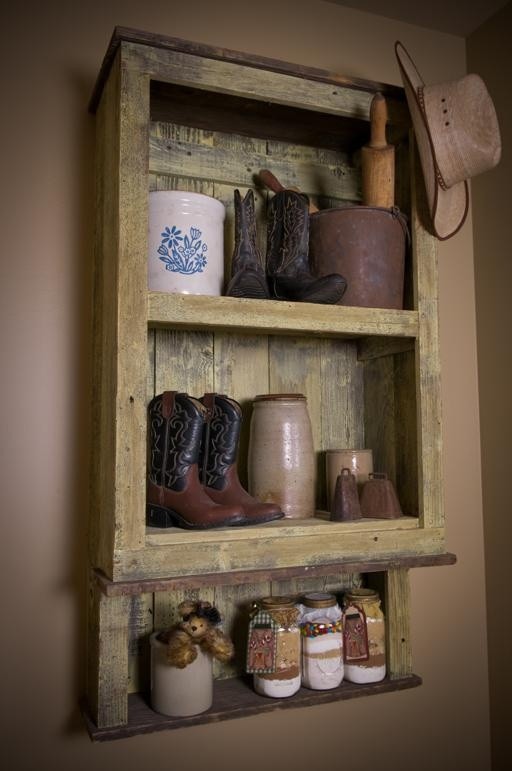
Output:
[83,36,451,581]
[79,573,423,738]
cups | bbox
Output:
[325,447,373,511]
[150,632,212,718]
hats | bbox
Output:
[394,37,503,241]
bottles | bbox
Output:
[248,394,317,519]
[250,589,385,698]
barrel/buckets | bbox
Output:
[309,203,409,310]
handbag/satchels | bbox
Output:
[329,469,404,522]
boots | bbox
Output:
[145,388,286,531]
[226,187,347,304]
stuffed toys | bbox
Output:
[161,600,234,669]
